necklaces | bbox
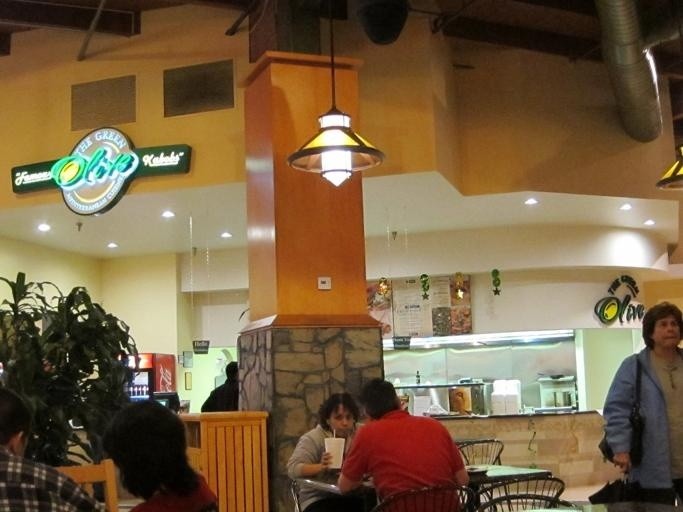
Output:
[650,346,681,390]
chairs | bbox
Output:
[288,478,301,511]
[478,496,577,512]
[459,440,510,512]
[372,485,475,512]
[53,460,119,512]
[475,477,566,512]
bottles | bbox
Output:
[126,384,148,394]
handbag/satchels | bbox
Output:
[598,360,646,468]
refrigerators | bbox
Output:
[122,353,176,402]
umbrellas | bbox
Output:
[589,460,646,505]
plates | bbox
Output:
[467,470,487,475]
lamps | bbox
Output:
[657,148,683,192]
[286,1,383,186]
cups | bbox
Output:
[324,437,346,471]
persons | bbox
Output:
[102,401,219,512]
[287,392,378,512]
[199,363,238,413]
[0,381,103,511]
[339,379,468,511]
[602,300,683,503]
